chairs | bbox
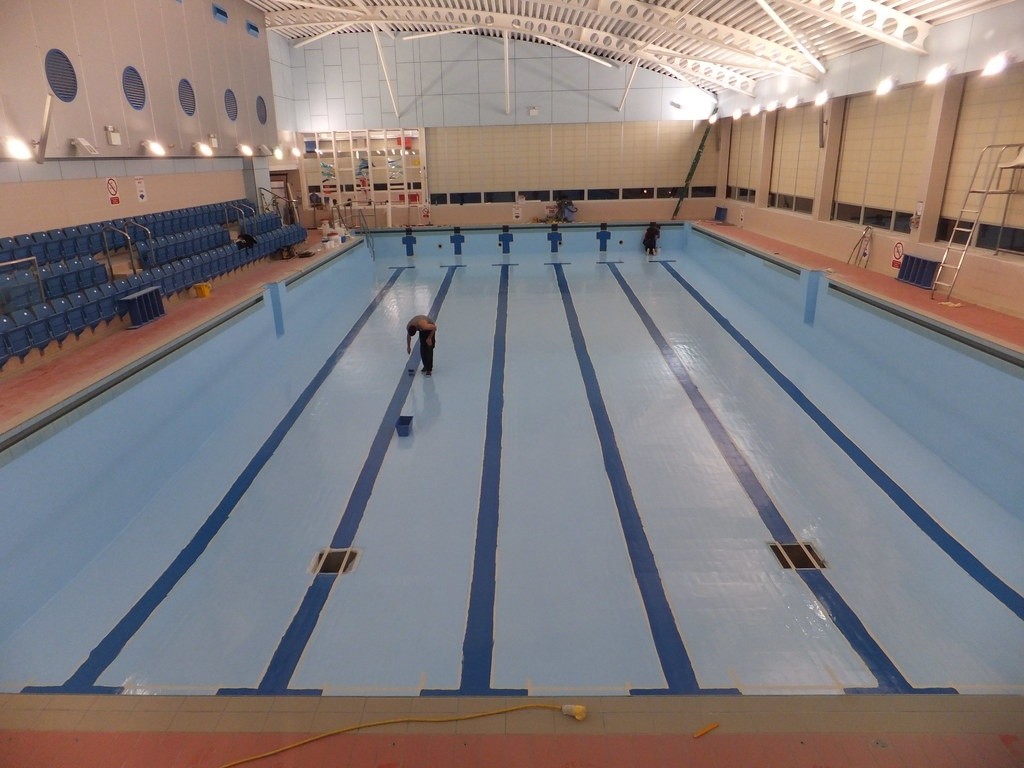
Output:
[0,199,307,371]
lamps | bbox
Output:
[209,134,217,148]
[192,142,213,157]
[71,137,99,156]
[529,106,539,116]
[235,144,253,157]
[105,125,122,145]
[141,140,164,155]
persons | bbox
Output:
[310,190,320,208]
[644,222,660,256]
[407,315,436,376]
[236,234,257,250]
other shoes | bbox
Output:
[652,248,656,256]
[426,371,431,377]
[646,248,650,254]
[421,367,427,373]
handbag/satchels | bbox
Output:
[280,245,295,260]
